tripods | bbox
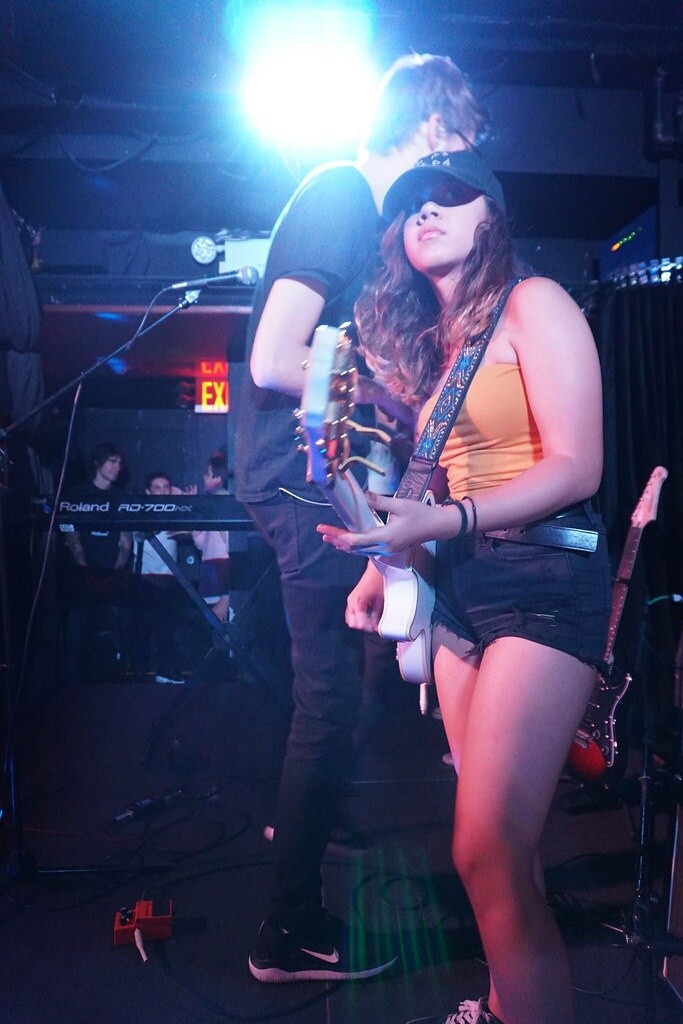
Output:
[560,601,683,1024]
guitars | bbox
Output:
[562,465,671,795]
[293,322,446,721]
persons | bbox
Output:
[231,51,499,984]
[316,149,612,1023]
[56,441,230,685]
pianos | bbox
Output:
[0,490,258,532]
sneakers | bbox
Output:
[248,909,402,983]
[266,807,381,857]
[404,997,500,1024]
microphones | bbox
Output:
[162,266,259,293]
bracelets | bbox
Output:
[461,496,478,540]
[441,498,468,541]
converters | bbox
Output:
[126,794,167,822]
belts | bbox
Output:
[479,526,598,555]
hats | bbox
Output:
[383,151,507,220]
